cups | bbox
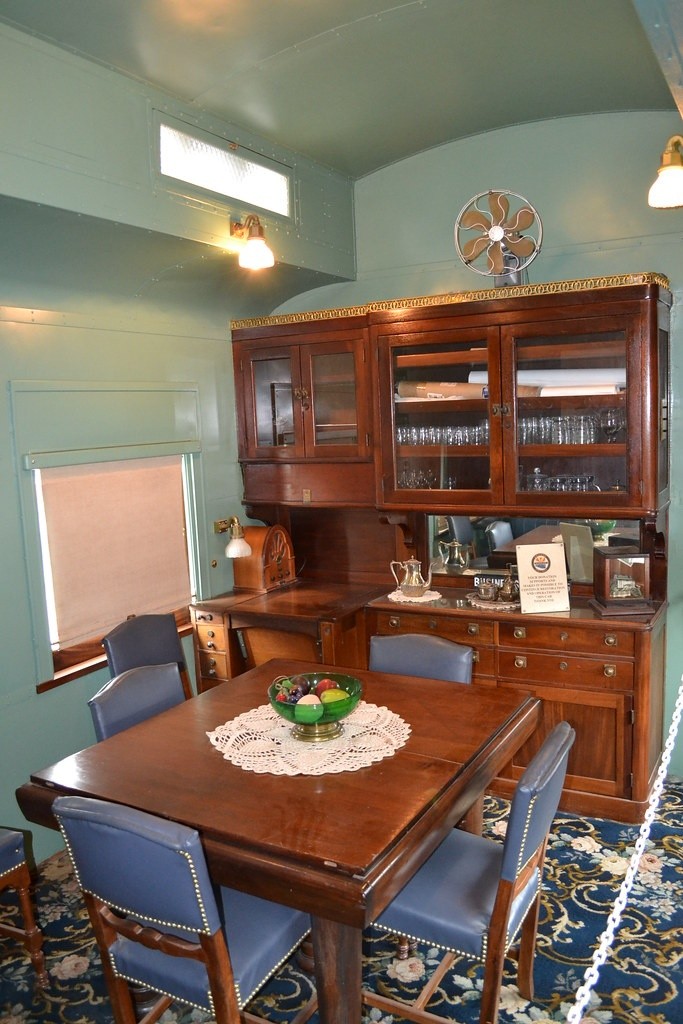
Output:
[395,415,599,446]
[517,467,596,493]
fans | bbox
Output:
[455,190,543,286]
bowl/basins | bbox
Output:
[477,581,500,600]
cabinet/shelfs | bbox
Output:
[190,273,673,822]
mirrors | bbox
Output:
[424,519,643,593]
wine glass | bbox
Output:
[599,407,624,444]
[396,467,456,489]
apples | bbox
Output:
[315,679,342,695]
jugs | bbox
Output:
[498,575,520,602]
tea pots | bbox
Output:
[389,556,437,598]
[438,539,474,575]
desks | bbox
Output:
[486,524,641,571]
[16,657,542,1024]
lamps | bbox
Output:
[648,136,683,208]
[214,515,251,558]
[230,213,274,270]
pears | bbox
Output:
[321,688,351,716]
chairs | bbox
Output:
[445,515,514,570]
[52,795,320,1024]
[0,826,50,988]
[364,721,576,1024]
[88,663,185,743]
[101,612,193,699]
[368,632,474,683]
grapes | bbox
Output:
[289,688,303,703]
